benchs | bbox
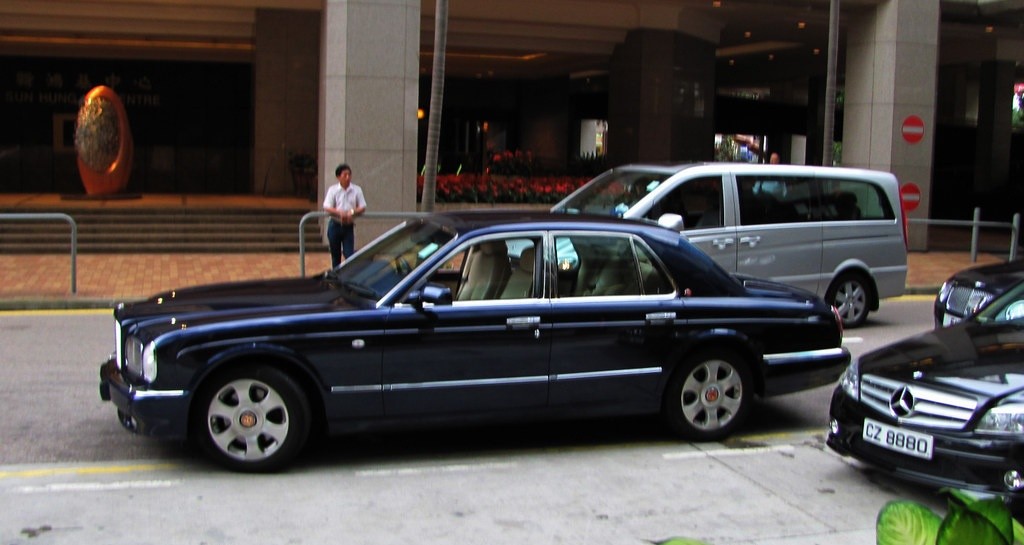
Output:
[575,254,658,297]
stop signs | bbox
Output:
[901,182,921,210]
[902,115,924,143]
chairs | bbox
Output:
[829,192,861,221]
[499,247,535,299]
[456,240,511,301]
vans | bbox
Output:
[547,162,908,327]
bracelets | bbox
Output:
[351,208,356,216]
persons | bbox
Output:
[323,163,366,270]
[752,151,788,203]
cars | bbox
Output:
[827,280,1024,509]
[933,257,1024,329]
[99,209,851,473]
